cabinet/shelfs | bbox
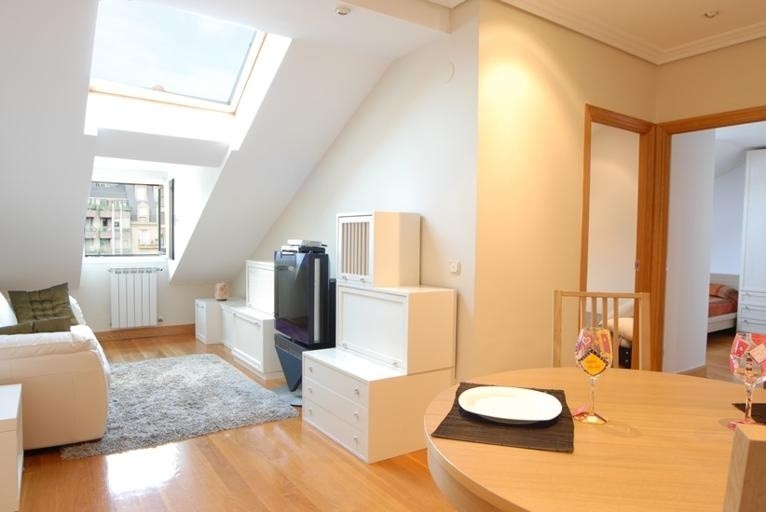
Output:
[301,345,457,466]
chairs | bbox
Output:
[552,289,652,373]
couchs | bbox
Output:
[0,285,113,451]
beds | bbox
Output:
[709,272,740,334]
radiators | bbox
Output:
[108,265,164,331]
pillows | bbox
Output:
[0,280,79,333]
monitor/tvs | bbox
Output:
[274,251,328,346]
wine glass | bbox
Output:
[571,326,613,425]
[727,332,766,430]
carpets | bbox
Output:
[55,352,299,458]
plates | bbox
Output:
[457,385,564,425]
